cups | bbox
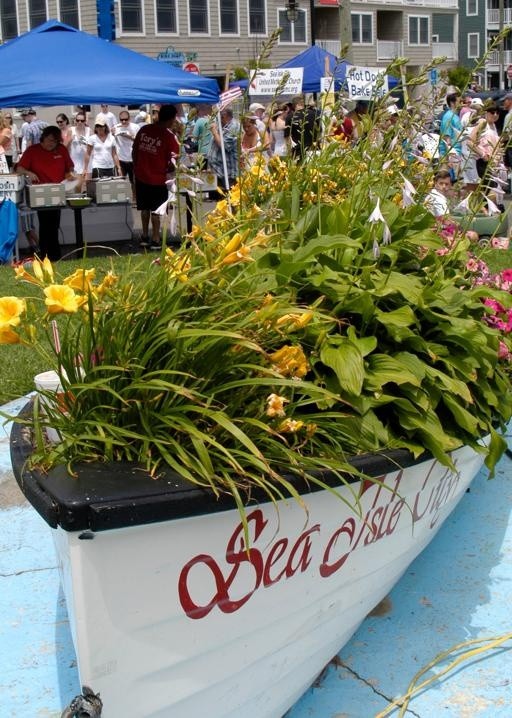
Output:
[33,365,89,446]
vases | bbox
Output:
[9,388,503,718]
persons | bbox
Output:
[1,93,512,264]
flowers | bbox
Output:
[0,0,512,563]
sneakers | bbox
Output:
[140,236,176,249]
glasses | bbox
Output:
[120,118,128,121]
[490,111,500,114]
[56,121,63,123]
[76,119,85,122]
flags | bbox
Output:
[217,85,243,112]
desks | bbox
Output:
[15,193,134,258]
[176,188,231,248]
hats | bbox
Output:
[249,102,266,114]
[385,96,404,116]
[470,98,484,108]
[95,116,106,126]
[500,92,512,101]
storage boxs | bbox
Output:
[25,183,66,208]
[0,172,24,192]
[176,172,217,192]
[86,179,132,204]
[0,192,22,204]
